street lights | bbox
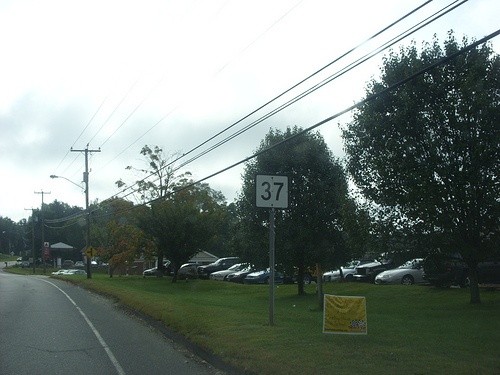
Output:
[50,174,91,280]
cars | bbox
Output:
[322,249,427,286]
[52,268,87,275]
[143,256,312,286]
